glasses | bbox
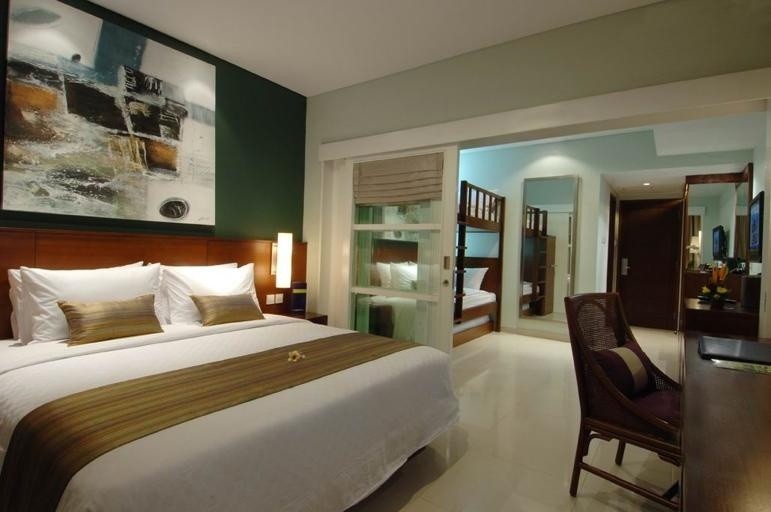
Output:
[688,234,699,270]
[270,232,294,289]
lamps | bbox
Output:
[562,293,682,511]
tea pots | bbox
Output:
[684,181,748,274]
[518,174,580,326]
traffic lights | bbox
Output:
[6,261,266,348]
[454,266,489,291]
[388,262,418,290]
[378,260,408,289]
[589,341,657,400]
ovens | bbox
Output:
[355,180,506,350]
[0,261,458,511]
[519,204,556,317]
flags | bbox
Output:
[710,300,725,310]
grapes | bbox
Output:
[282,312,329,326]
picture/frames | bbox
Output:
[685,296,759,338]
[685,330,770,512]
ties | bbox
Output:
[748,190,764,263]
[712,225,727,261]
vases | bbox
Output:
[287,350,304,363]
[697,266,730,302]
